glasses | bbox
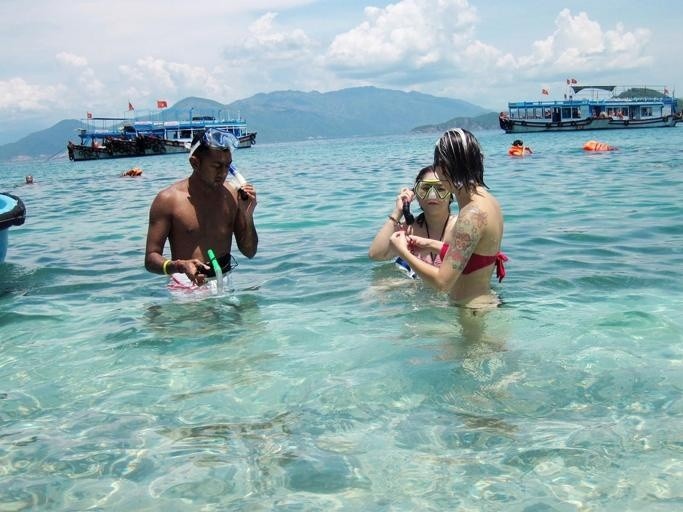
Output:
[201,127,241,153]
[412,178,453,201]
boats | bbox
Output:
[0,189,27,270]
[67,97,258,161]
[498,78,682,134]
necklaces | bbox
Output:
[422,217,448,266]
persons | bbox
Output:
[508,139,532,158]
[368,165,459,286]
[25,175,32,184]
[499,112,509,123]
[143,125,260,306]
[389,127,508,308]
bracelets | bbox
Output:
[175,259,180,273]
[162,260,172,275]
[387,215,398,224]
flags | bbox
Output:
[664,89,669,94]
[572,79,577,83]
[87,113,91,119]
[157,101,167,109]
[567,79,570,85]
[128,102,134,112]
[542,89,548,95]
